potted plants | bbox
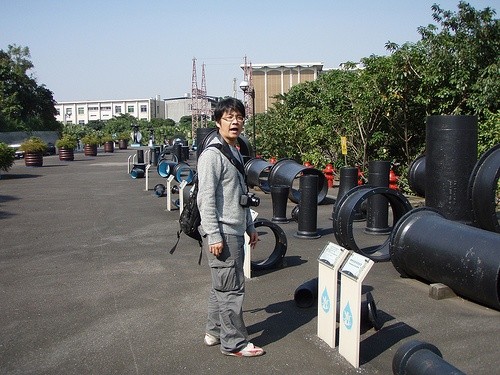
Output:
[117,133,131,149]
[101,134,116,152]
[56,135,77,160]
[82,134,100,156]
[20,136,48,167]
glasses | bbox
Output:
[220,116,246,123]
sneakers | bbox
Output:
[222,340,265,357]
[204,334,220,346]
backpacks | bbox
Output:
[178,182,203,242]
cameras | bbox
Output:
[240,192,260,208]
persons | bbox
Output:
[178,99,265,357]
